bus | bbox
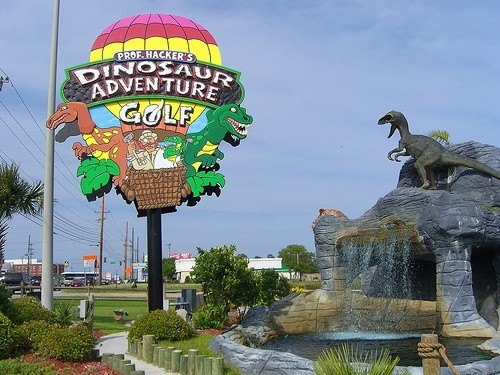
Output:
[61,271,100,284]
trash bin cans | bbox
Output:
[79,300,88,319]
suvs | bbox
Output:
[4,272,35,295]
[26,276,45,297]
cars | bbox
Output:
[100,278,117,285]
[71,279,83,287]
[63,277,74,286]
[84,278,98,286]
